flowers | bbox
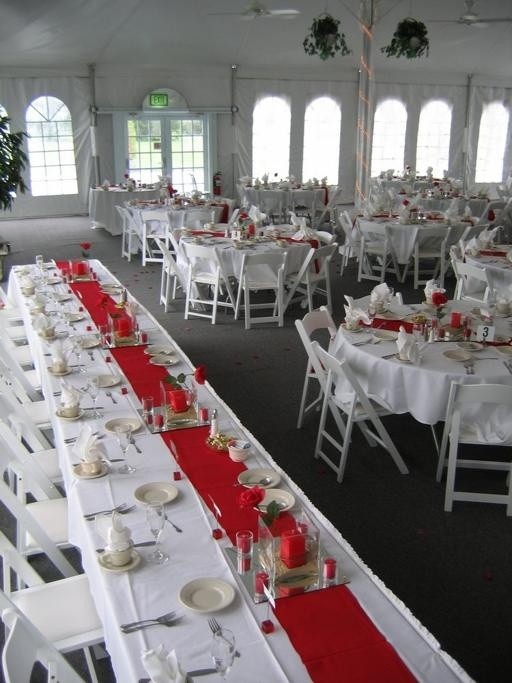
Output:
[79,242,96,260]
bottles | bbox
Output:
[208,408,219,438]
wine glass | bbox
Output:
[211,629,236,683]
[87,376,103,419]
[116,425,136,474]
[144,501,168,563]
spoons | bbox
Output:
[233,477,272,488]
[255,502,287,510]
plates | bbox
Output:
[87,375,122,388]
[177,577,236,613]
[104,416,142,434]
[18,252,181,376]
[97,551,141,573]
[55,408,86,421]
[238,468,295,513]
[72,464,109,478]
[340,286,512,362]
[134,482,178,507]
[190,217,301,249]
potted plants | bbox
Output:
[301,11,352,61]
[376,17,430,61]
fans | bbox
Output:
[206,0,300,21]
[428,0,512,28]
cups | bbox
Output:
[141,377,211,432]
[80,458,102,474]
[100,540,133,566]
[227,440,251,463]
[237,527,337,601]
[59,401,80,418]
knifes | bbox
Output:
[63,430,105,446]
[73,459,125,466]
[95,540,160,554]
[139,669,218,683]
[84,407,104,410]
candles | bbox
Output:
[280,529,308,568]
[255,573,269,593]
[92,272,96,279]
[238,533,251,553]
[323,559,335,579]
[66,275,72,282]
[74,263,85,275]
[239,558,250,571]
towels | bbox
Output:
[92,512,132,551]
[139,644,188,683]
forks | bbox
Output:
[105,391,116,403]
[120,611,184,633]
[129,437,142,453]
[157,509,183,533]
[84,502,137,521]
[208,617,240,657]
